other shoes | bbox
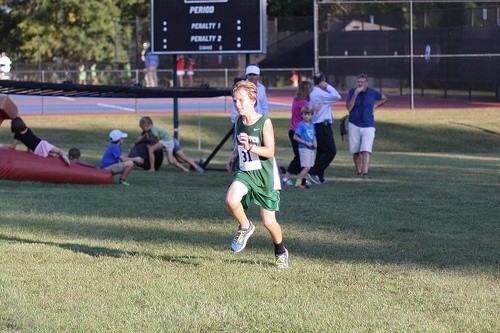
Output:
[357,172,371,177]
[196,167,204,172]
[119,177,130,185]
[301,181,310,188]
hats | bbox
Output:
[108,130,128,142]
[245,66,260,75]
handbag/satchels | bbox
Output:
[340,115,349,136]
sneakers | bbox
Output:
[293,184,305,189]
[231,221,255,252]
[275,246,288,269]
[281,178,294,186]
[308,173,321,184]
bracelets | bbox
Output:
[247,147,253,152]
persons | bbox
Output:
[308,72,341,185]
[176,55,185,87]
[90,63,97,85]
[78,64,87,85]
[225,80,288,270]
[8,117,71,166]
[346,74,389,179]
[230,65,268,171]
[131,117,205,173]
[187,55,195,87]
[290,71,299,87]
[0,51,13,80]
[99,130,134,186]
[293,106,318,190]
[280,81,321,186]
[141,42,159,87]
